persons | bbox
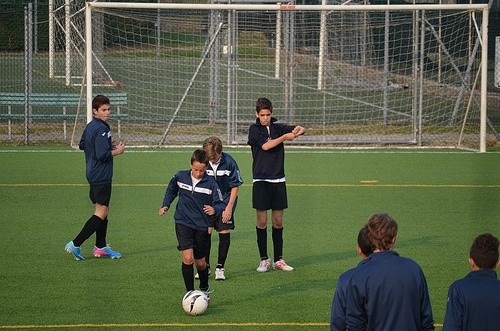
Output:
[330,226,377,331]
[247,97,306,272]
[442,233,500,331]
[158,149,225,301]
[194,136,243,280]
[65,95,125,261]
[346,213,434,331]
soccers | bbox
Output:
[182,289,208,315]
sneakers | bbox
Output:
[199,288,211,303]
[257,259,271,272]
[64,240,85,260]
[214,268,226,280]
[272,259,294,272]
[92,242,122,259]
[194,264,212,279]
